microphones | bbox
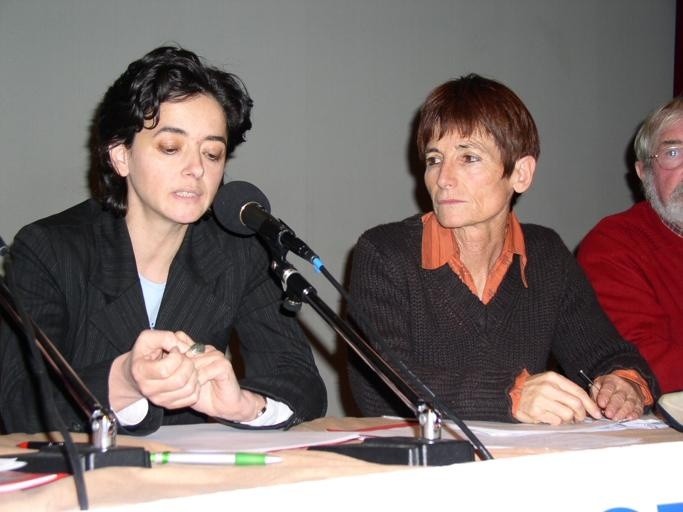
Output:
[211,179,324,270]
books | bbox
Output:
[140,419,358,455]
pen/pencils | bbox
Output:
[579,370,591,385]
[150,451,287,466]
[16,440,51,449]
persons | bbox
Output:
[0,41,329,440]
[573,99,682,416]
[341,70,665,426]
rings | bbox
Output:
[189,342,206,356]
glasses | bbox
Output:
[642,144,683,171]
